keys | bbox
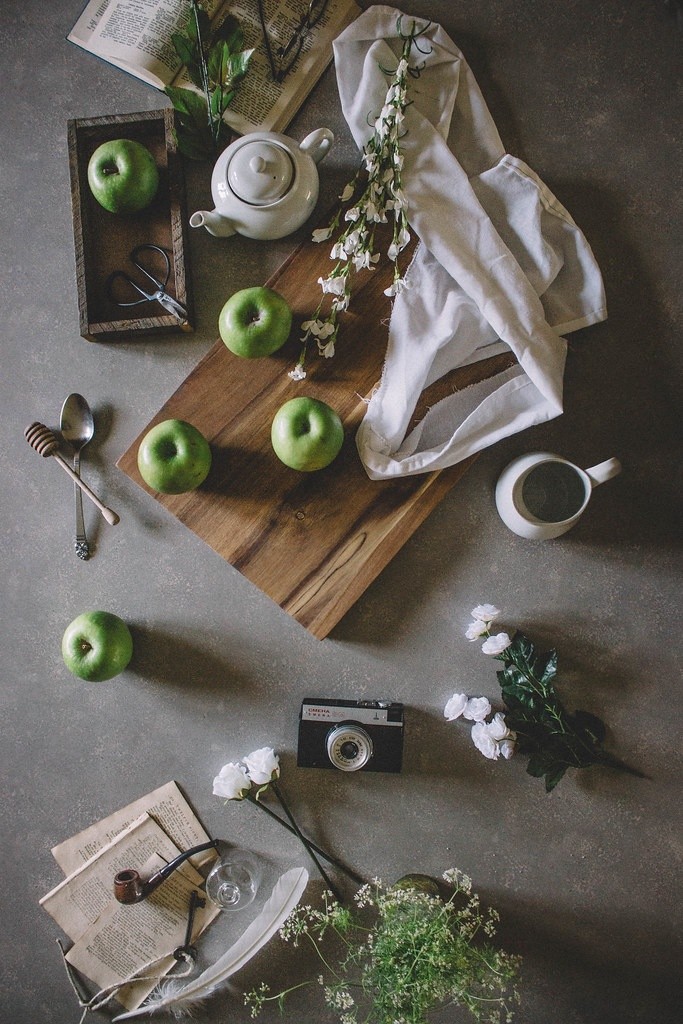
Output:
[174,891,206,962]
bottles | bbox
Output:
[206,848,262,909]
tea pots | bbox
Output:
[190,128,335,240]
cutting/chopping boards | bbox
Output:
[117,205,516,641]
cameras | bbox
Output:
[297,698,404,773]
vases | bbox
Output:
[389,873,441,920]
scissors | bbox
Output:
[105,244,188,321]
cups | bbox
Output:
[494,452,622,541]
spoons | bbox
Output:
[59,393,94,560]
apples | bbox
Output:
[272,396,344,472]
[88,138,158,215]
[217,286,291,360]
[62,610,132,682]
[138,418,211,497]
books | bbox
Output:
[65,0,363,137]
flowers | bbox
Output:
[208,745,369,901]
[286,7,432,383]
[439,602,652,801]
[230,866,524,1024]
